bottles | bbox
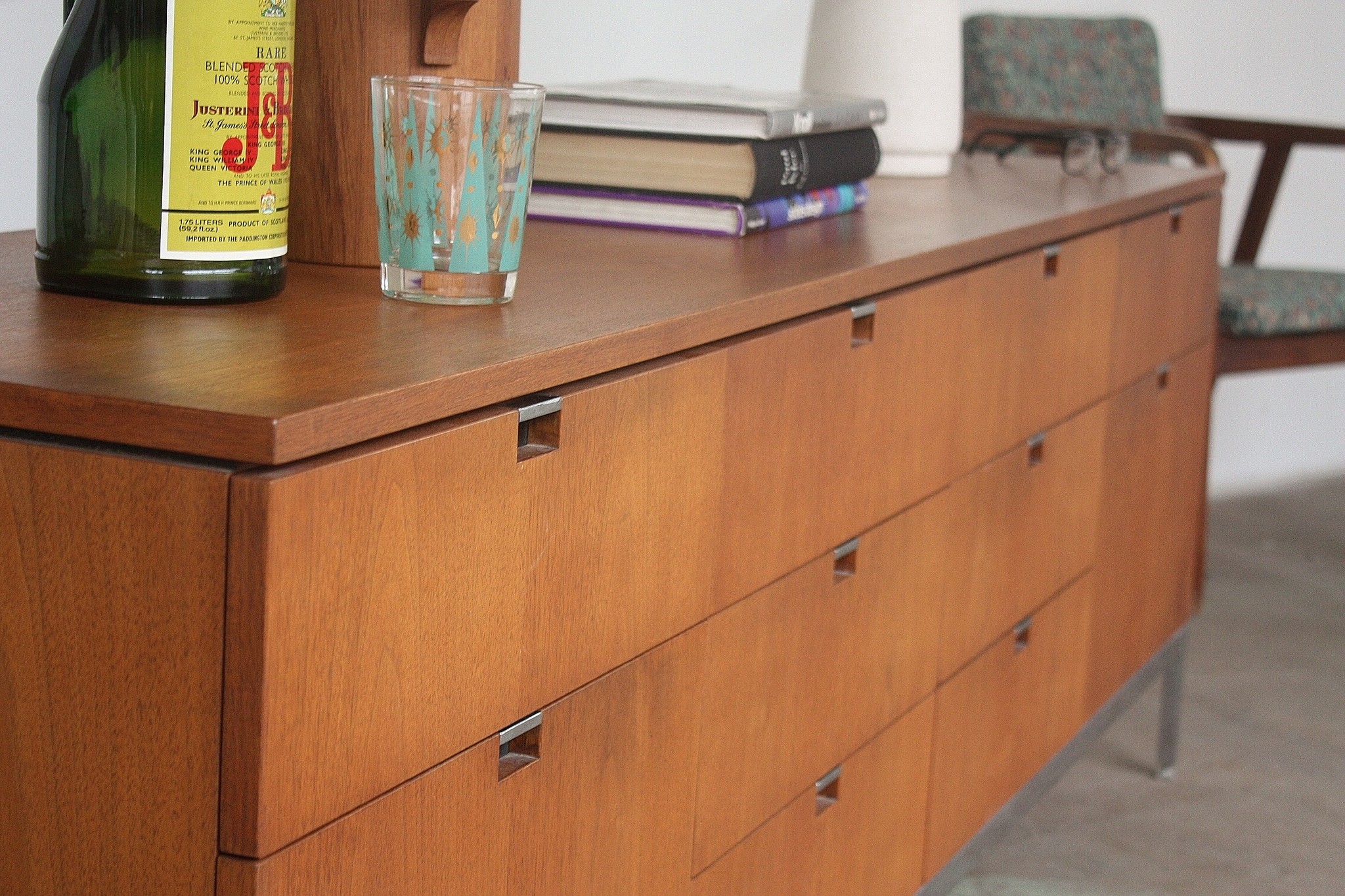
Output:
[36,1,295,303]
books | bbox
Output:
[499,180,869,239]
[504,123,882,203]
[508,78,886,139]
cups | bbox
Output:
[369,74,544,307]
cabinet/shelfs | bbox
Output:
[0,170,1228,895]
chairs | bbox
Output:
[956,9,1345,602]
